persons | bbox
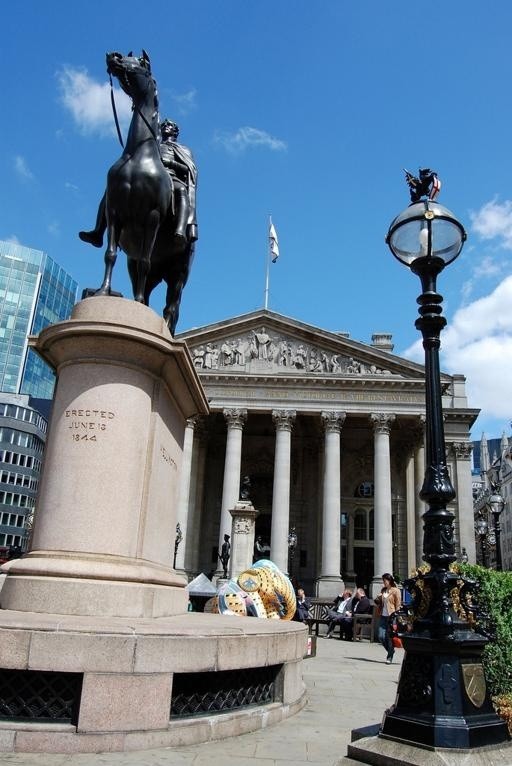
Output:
[191,325,393,376]
[75,116,199,248]
[219,533,229,579]
[377,573,401,663]
[253,534,269,561]
[323,589,352,640]
[295,589,311,623]
[343,588,370,640]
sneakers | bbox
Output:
[385,660,391,664]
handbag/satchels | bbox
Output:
[392,623,403,647]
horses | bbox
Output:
[92,48,195,338]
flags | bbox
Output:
[268,214,280,264]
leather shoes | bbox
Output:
[324,634,331,639]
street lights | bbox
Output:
[473,508,491,570]
[487,483,506,572]
[173,521,183,568]
[288,525,297,581]
[379,159,511,750]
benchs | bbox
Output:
[298,602,377,641]
